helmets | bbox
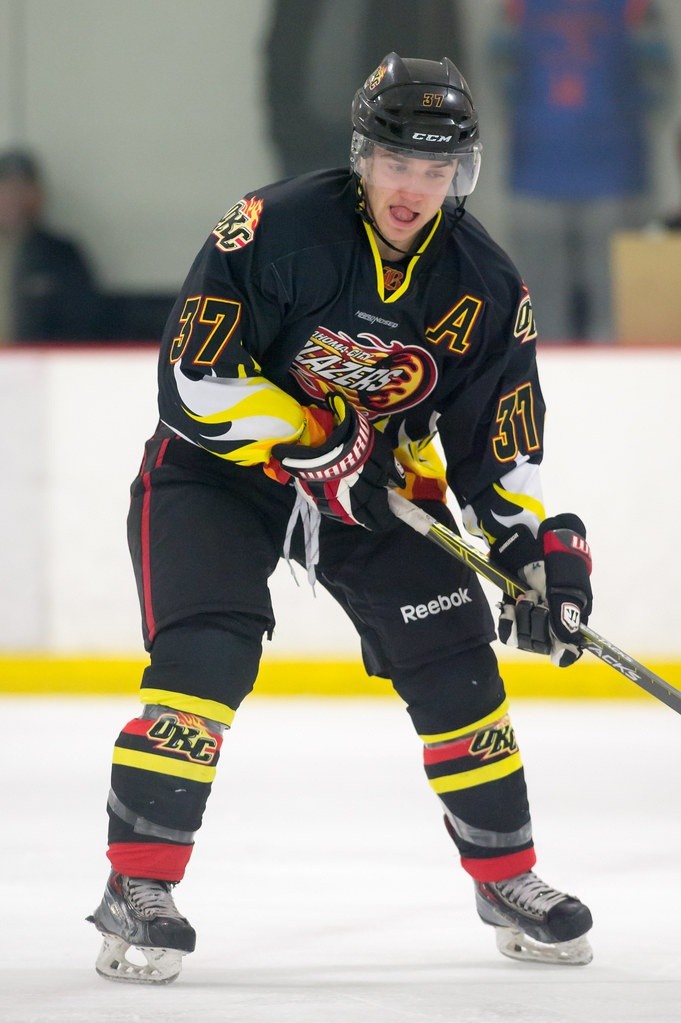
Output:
[346,51,480,169]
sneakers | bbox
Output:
[85,869,196,985]
[475,870,596,965]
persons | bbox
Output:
[1,150,104,345]
[89,54,609,953]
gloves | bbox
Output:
[270,392,409,534]
[489,513,595,669]
